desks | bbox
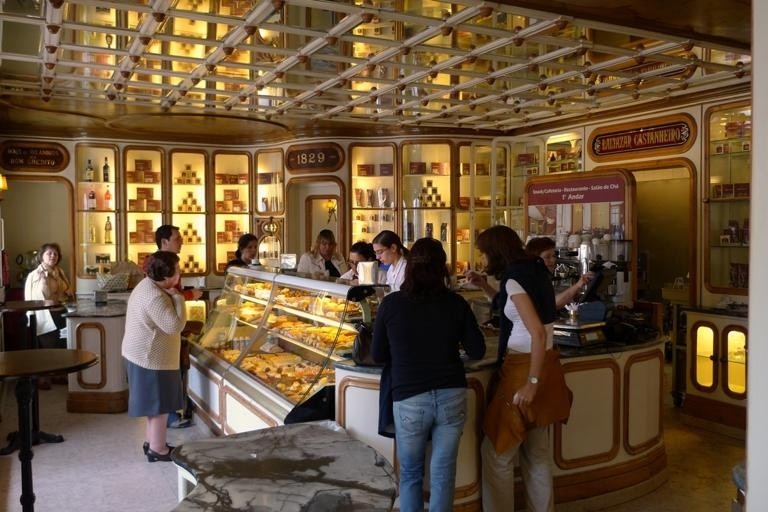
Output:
[1,348,101,512]
[169,424,401,512]
[0,299,67,457]
[64,300,128,414]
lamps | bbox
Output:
[324,200,337,224]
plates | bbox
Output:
[460,283,480,290]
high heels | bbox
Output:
[147,445,177,462]
[143,441,168,455]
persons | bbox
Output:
[350,229,410,289]
[23,242,69,392]
[336,240,381,287]
[223,231,259,280]
[119,249,188,463]
[524,235,599,312]
[464,225,574,510]
[295,227,352,280]
[154,224,204,430]
[369,237,487,511]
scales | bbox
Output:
[553,322,607,347]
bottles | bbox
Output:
[88,217,95,242]
[548,153,556,172]
[104,215,112,243]
[85,160,94,181]
[104,184,112,209]
[102,155,109,180]
[204,330,252,355]
[263,332,273,344]
[89,186,96,209]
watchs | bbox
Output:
[527,375,540,385]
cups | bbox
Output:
[554,224,623,300]
[319,269,328,280]
[251,259,259,265]
[487,276,500,301]
[357,261,378,285]
[279,254,296,270]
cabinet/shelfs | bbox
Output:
[545,131,584,173]
[185,265,390,438]
[166,148,210,277]
[209,150,253,275]
[122,144,166,272]
[73,142,122,281]
[662,283,684,408]
[348,142,399,249]
[702,96,752,299]
[254,146,286,218]
[456,140,508,210]
[509,140,542,206]
[679,306,746,433]
[453,209,507,277]
[400,139,455,253]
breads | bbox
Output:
[217,282,378,405]
